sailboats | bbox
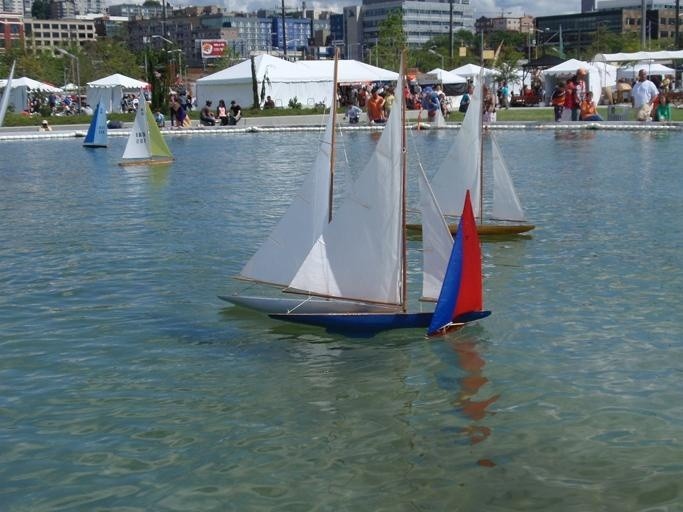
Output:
[118,86,177,167]
[82,94,110,150]
[406,63,535,236]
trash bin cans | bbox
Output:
[607,105,629,121]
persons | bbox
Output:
[200,99,242,126]
[631,69,672,122]
[155,79,192,128]
[262,96,274,108]
[28,93,91,116]
[460,81,511,122]
[522,74,544,104]
[337,84,448,123]
[121,94,137,113]
[552,67,604,121]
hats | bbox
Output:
[423,86,432,94]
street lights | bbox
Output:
[151,34,176,65]
[54,47,83,121]
[428,49,445,70]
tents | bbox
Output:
[425,64,533,111]
[542,58,675,106]
[196,55,334,110]
[85,74,150,114]
